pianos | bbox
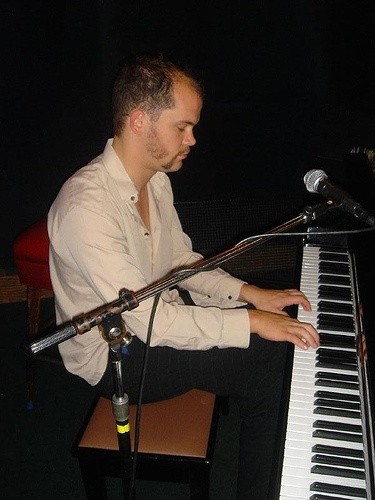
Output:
[278,224,375,498]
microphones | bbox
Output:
[304,169,375,226]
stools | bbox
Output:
[71,388,231,500]
[12,214,51,412]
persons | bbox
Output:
[46,55,320,500]
